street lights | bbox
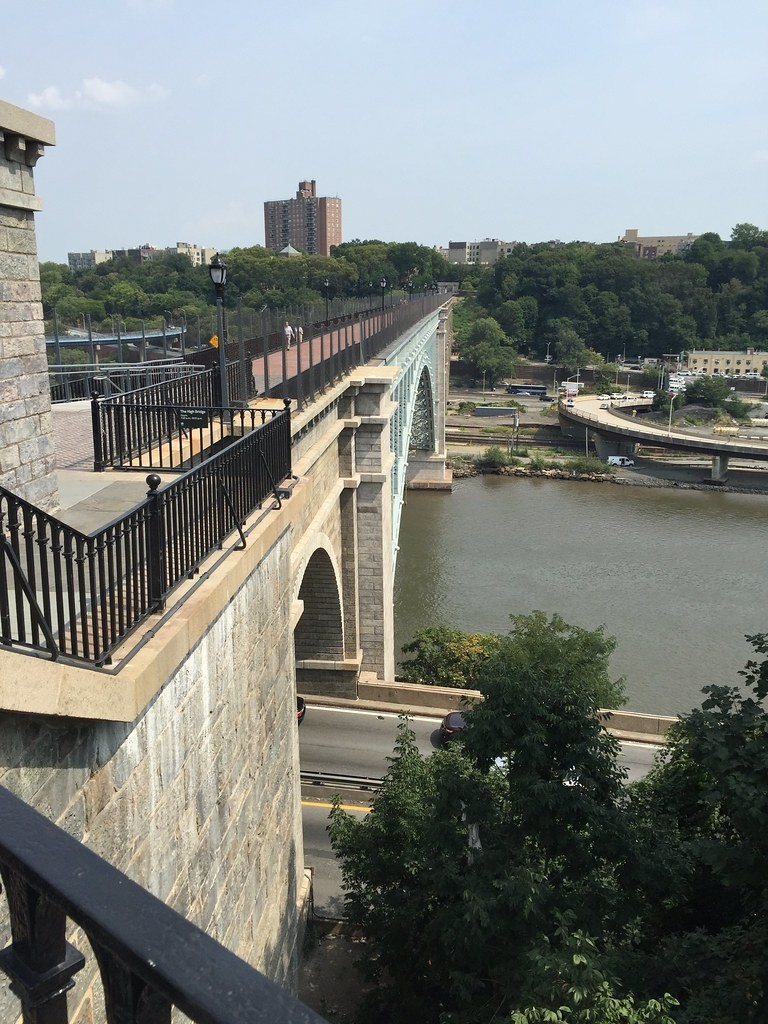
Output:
[368,281,372,312]
[323,278,330,322]
[390,283,394,308]
[408,281,412,302]
[208,254,232,423]
[381,277,386,311]
[424,283,427,297]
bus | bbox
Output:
[507,384,547,396]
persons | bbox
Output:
[284,321,293,350]
[400,297,408,306]
[295,323,303,343]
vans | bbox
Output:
[606,456,635,468]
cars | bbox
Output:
[540,395,554,402]
[558,366,762,409]
[440,712,467,744]
[297,694,306,725]
